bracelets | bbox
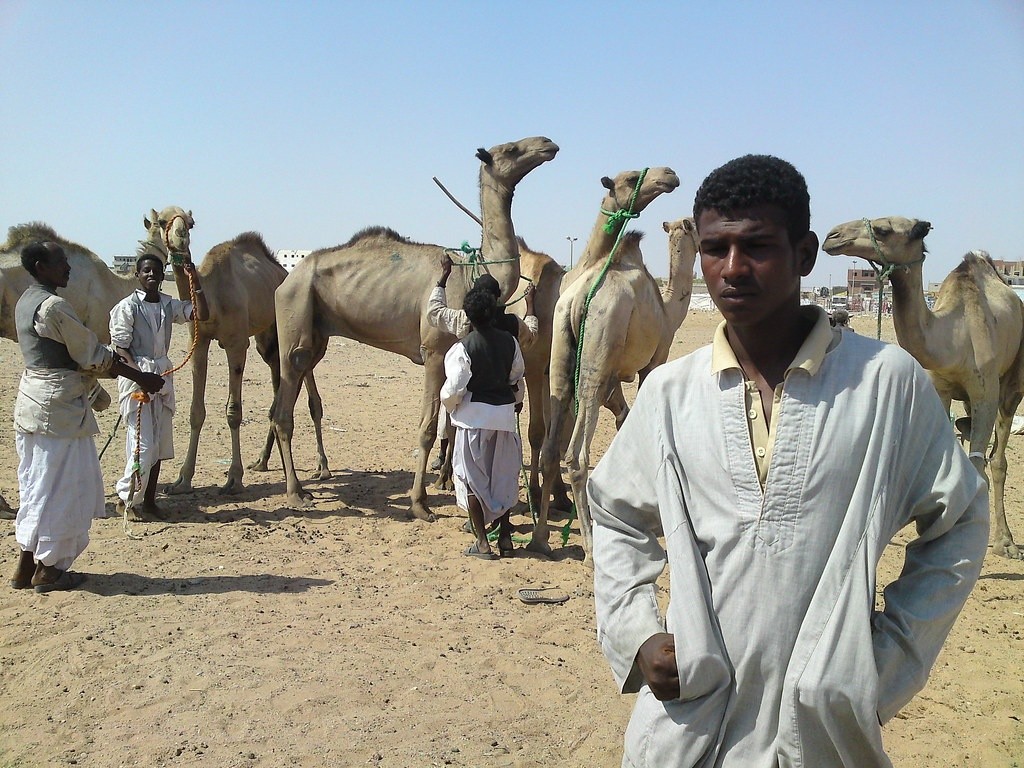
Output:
[194,287,204,294]
[437,281,446,288]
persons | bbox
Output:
[108,253,214,522]
[11,240,168,593]
[585,151,988,767]
[425,253,539,560]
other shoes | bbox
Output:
[517,587,570,602]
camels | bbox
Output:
[1,134,1024,559]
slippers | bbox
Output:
[34,570,90,593]
[496,540,514,557]
[457,521,473,532]
[11,564,37,588]
[464,540,497,560]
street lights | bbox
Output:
[566,236,577,270]
[851,260,857,310]
